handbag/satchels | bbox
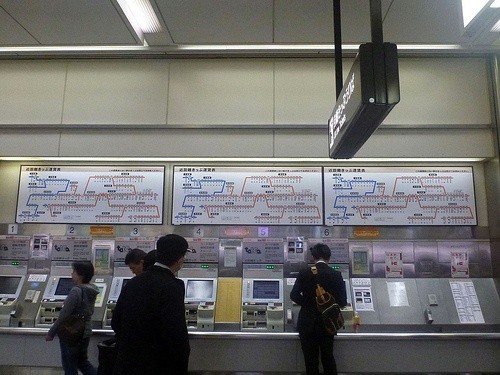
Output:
[56,286,86,340]
[316,292,344,334]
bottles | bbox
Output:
[351,312,360,333]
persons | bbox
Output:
[289,243,347,375]
[110,234,191,375]
[123,247,179,279]
[46,259,101,375]
